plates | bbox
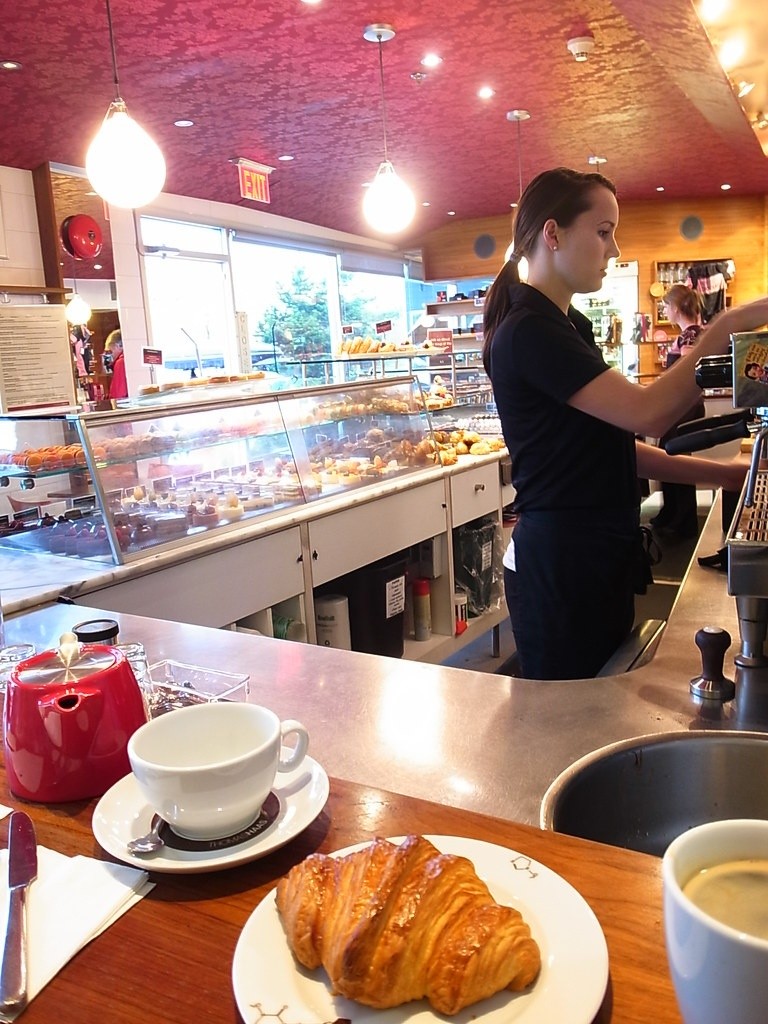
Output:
[92,746,331,873]
[231,834,609,1024]
[650,282,664,297]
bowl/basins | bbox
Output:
[539,730,768,858]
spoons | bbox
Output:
[127,812,164,852]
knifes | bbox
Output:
[0,810,39,1015]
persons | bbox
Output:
[649,285,705,539]
[102,328,133,437]
[481,166,768,680]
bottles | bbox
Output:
[658,262,688,320]
[315,595,351,650]
[412,577,433,640]
[76,378,85,402]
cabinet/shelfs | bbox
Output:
[422,297,496,408]
[0,375,519,664]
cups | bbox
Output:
[0,619,152,698]
[128,702,309,839]
[662,818,768,1024]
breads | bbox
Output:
[276,831,542,1016]
[140,371,264,396]
[0,391,506,555]
[337,336,435,354]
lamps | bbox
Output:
[505,109,528,283]
[64,258,92,324]
[363,23,416,235]
[85,0,166,209]
[749,112,768,129]
[730,79,755,98]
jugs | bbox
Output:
[3,631,149,804]
